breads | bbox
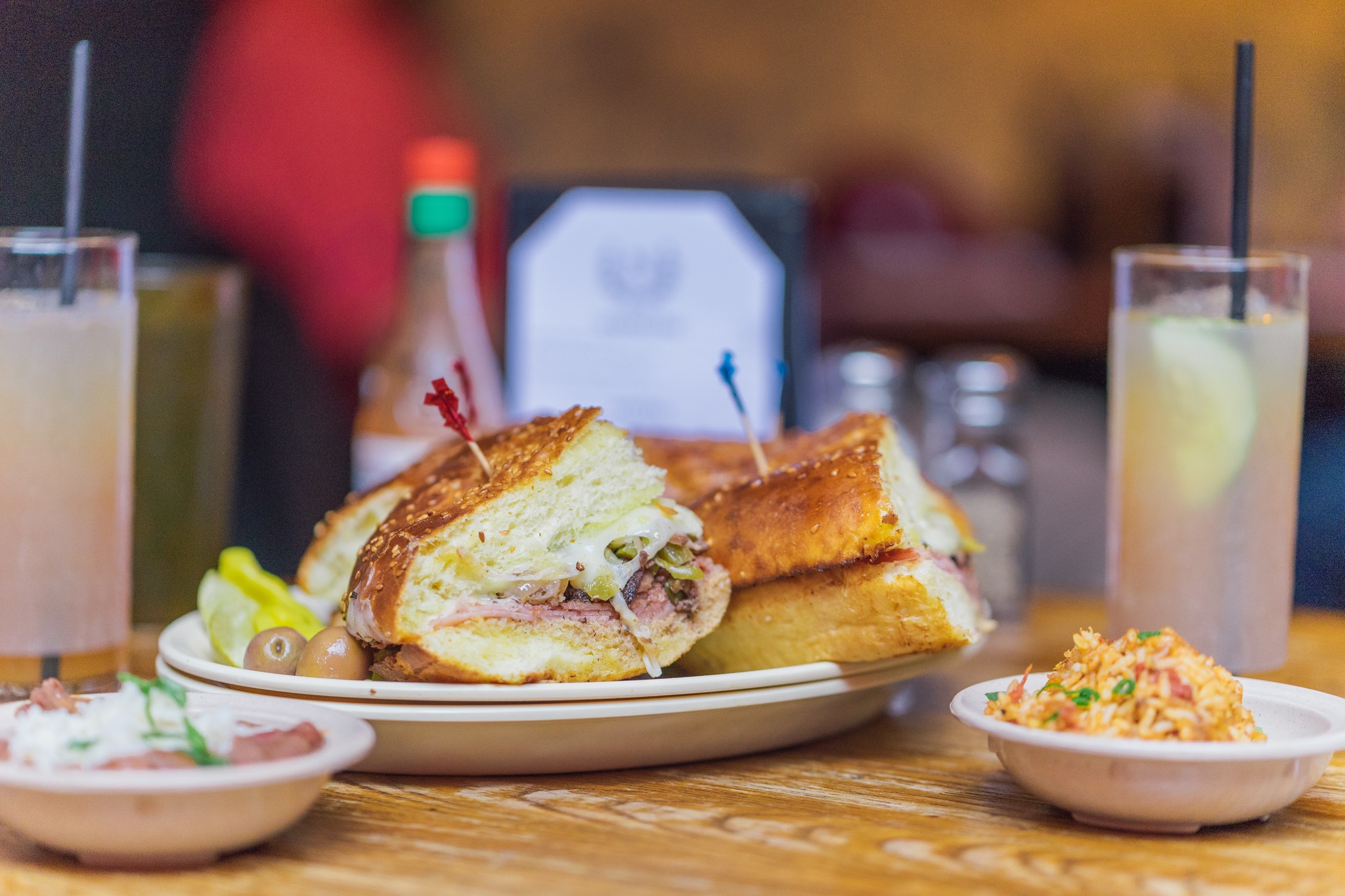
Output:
[294,402,977,683]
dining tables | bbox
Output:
[0,595,1343,895]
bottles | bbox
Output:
[346,137,505,505]
[925,335,1037,623]
[806,342,908,440]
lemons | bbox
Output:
[197,548,330,668]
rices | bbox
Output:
[984,627,1268,745]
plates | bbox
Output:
[0,686,378,866]
[954,663,1344,835]
[153,590,999,784]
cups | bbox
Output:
[1107,237,1308,674]
[134,249,253,626]
[1,215,140,698]
